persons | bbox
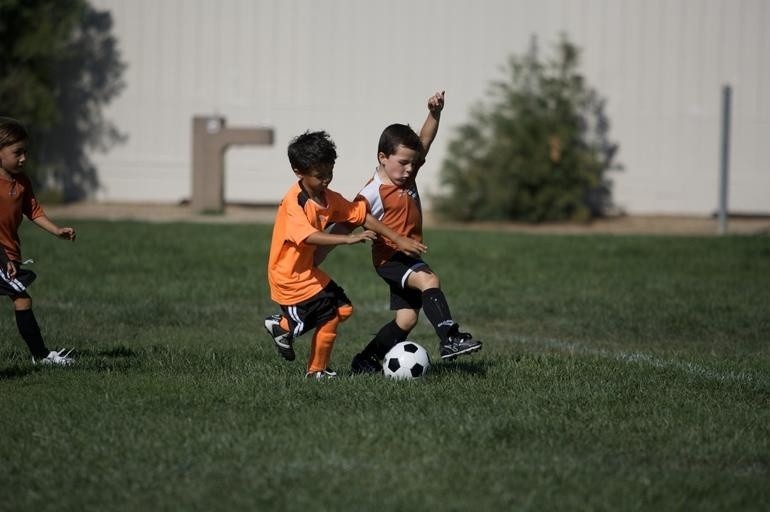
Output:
[312,87,482,378]
[1,114,81,373]
[261,126,429,385]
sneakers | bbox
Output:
[439,332,483,361]
[31,348,77,367]
[305,367,338,379]
[351,353,383,376]
[264,313,296,362]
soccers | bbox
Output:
[382,340,432,381]
